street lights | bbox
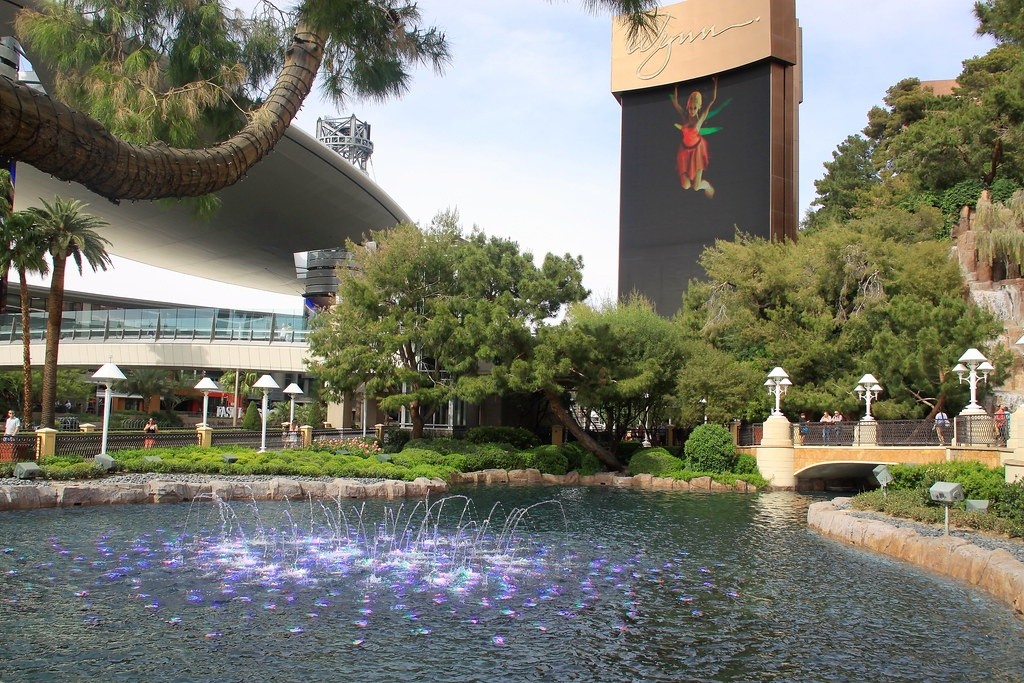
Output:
[91,363,127,454]
[357,382,377,439]
[282,383,303,432]
[193,377,218,426]
[952,348,995,446]
[252,375,281,454]
[760,367,794,447]
[852,373,884,447]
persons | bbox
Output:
[992,404,1011,447]
[932,406,949,446]
[663,75,720,199]
[281,420,299,450]
[2,408,20,442]
[799,413,810,446]
[143,417,159,450]
[820,412,831,446]
[650,419,667,446]
[830,410,843,446]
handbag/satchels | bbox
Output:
[944,420,950,427]
[801,427,809,433]
